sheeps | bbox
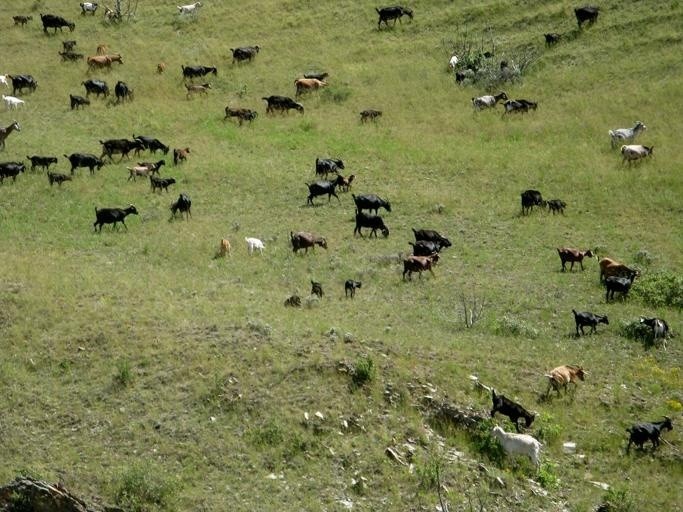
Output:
[219,236,266,257]
[490,390,543,475]
[445,50,539,118]
[63,152,107,175]
[625,415,674,456]
[25,154,58,174]
[0,0,136,111]
[181,44,330,126]
[176,0,201,19]
[0,161,28,183]
[92,133,192,232]
[608,120,654,169]
[543,7,599,48]
[520,189,566,215]
[285,157,451,308]
[543,363,588,398]
[0,118,23,151]
[155,62,167,76]
[375,5,414,30]
[46,170,73,190]
[359,109,383,124]
[556,247,670,349]
